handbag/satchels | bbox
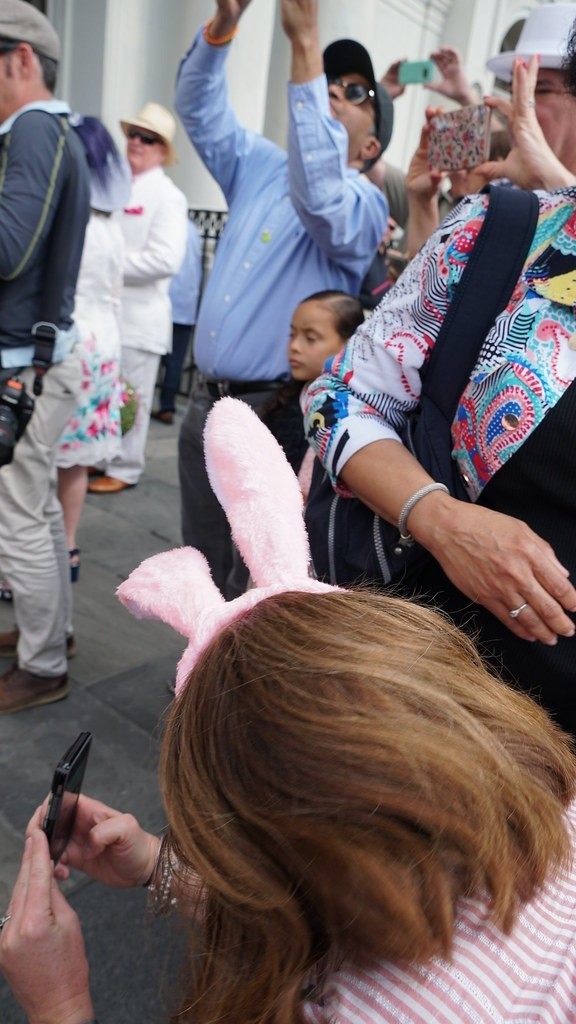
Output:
[307,184,540,599]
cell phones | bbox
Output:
[398,60,433,84]
[42,732,95,870]
[428,104,492,171]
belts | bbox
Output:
[201,378,286,401]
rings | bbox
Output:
[510,602,528,618]
[0,915,11,928]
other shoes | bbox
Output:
[87,475,134,494]
[0,588,13,602]
[87,466,106,476]
[0,629,76,659]
[0,663,72,714]
[149,409,174,424]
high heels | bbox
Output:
[68,549,81,583]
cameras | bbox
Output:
[0,371,35,467]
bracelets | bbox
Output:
[205,19,236,43]
[394,483,449,553]
[144,837,177,914]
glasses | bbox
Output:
[126,128,167,152]
[325,75,378,117]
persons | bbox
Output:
[0,116,130,601]
[174,0,394,598]
[0,593,576,1024]
[271,20,576,475]
[302,18,576,746]
[87,102,188,493]
[0,0,91,715]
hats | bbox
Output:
[0,0,62,63]
[322,38,394,174]
[486,4,576,86]
[119,101,178,168]
[70,111,132,212]
